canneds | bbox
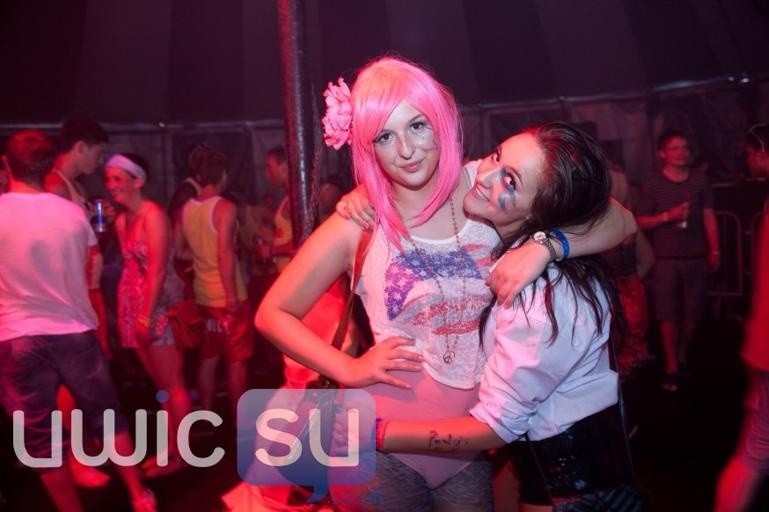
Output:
[676,201,689,230]
[93,199,108,232]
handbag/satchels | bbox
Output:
[553,486,647,512]
[164,297,207,351]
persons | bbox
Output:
[0,55,769,512]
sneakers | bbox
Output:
[73,449,191,512]
